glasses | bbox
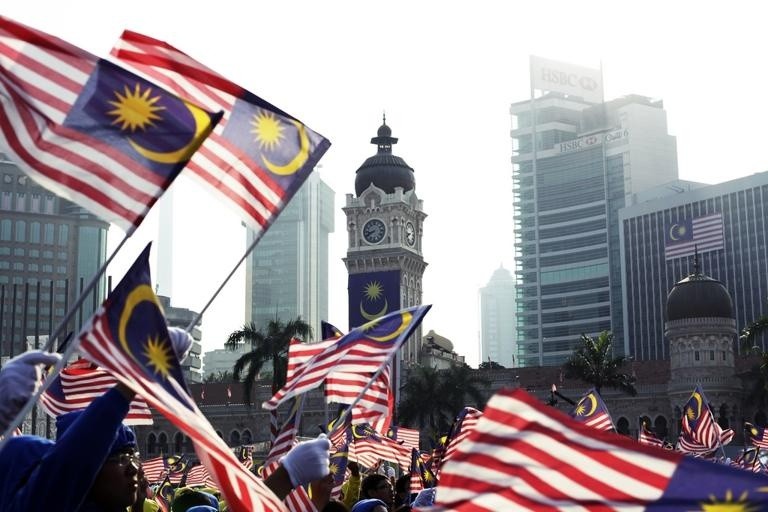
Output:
[107,451,141,467]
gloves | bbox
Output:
[0,350,62,417]
[279,433,331,488]
[166,326,193,365]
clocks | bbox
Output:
[404,221,416,248]
[361,217,389,246]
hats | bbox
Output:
[55,410,137,449]
[412,488,436,509]
[351,498,388,512]
[187,491,219,512]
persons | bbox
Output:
[0,324,197,511]
[0,348,64,434]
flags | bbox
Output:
[0,13,225,238]
[107,26,330,232]
